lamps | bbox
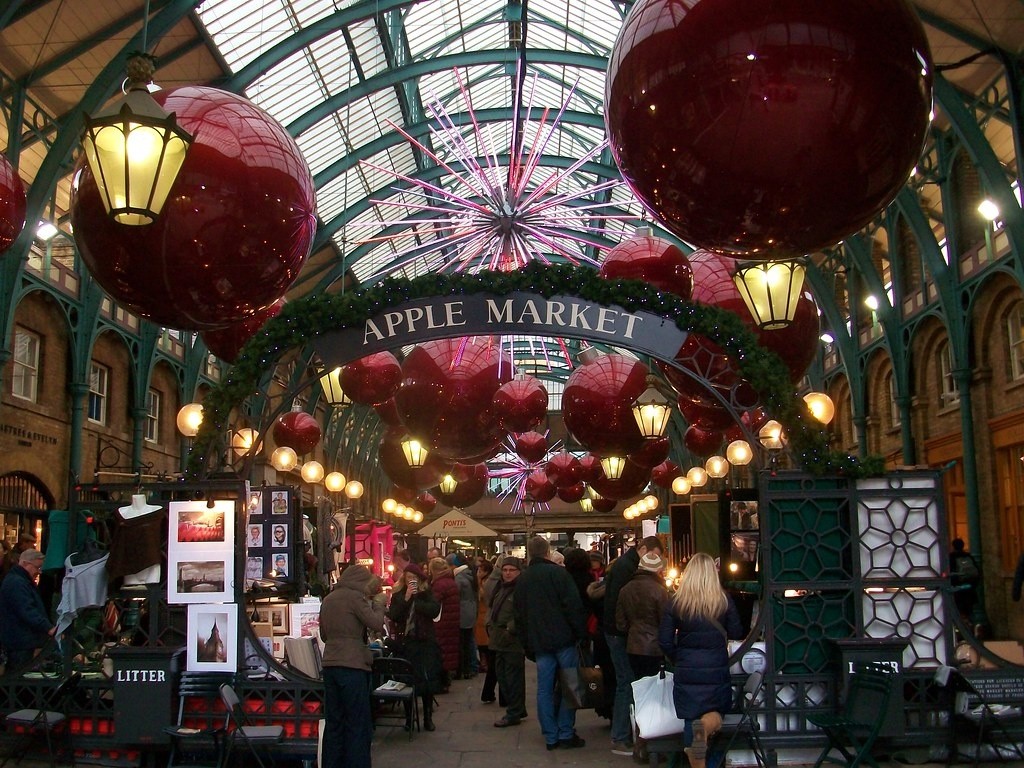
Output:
[729,258,808,330]
[79,0,195,225]
[317,340,676,515]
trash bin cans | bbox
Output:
[105,648,186,744]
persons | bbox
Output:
[743,540,759,580]
[485,557,528,727]
[319,564,387,768]
[111,495,169,585]
[0,540,10,563]
[11,533,36,563]
[513,536,588,751]
[0,548,56,675]
[417,542,606,707]
[603,535,664,757]
[389,565,442,731]
[56,538,112,650]
[732,503,751,530]
[614,551,669,679]
[949,538,983,640]
[659,552,743,768]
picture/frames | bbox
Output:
[167,500,237,673]
[245,602,290,635]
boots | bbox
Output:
[682,748,706,768]
[403,702,415,731]
[691,711,722,760]
[422,705,435,731]
[478,652,489,672]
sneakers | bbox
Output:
[546,741,559,751]
[560,734,585,748]
[610,740,634,756]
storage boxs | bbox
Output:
[284,636,324,679]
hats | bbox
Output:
[22,534,35,543]
[501,557,522,571]
[527,535,549,558]
[590,551,603,562]
[403,564,428,582]
[637,552,663,572]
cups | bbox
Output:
[409,581,418,594]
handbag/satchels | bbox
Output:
[629,657,685,739]
[561,645,611,710]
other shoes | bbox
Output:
[519,711,528,718]
[481,697,496,701]
[494,716,520,727]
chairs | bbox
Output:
[219,683,285,768]
[370,637,439,742]
[0,672,81,768]
[936,665,1024,768]
[161,671,237,768]
[804,663,894,768]
[705,671,770,768]
